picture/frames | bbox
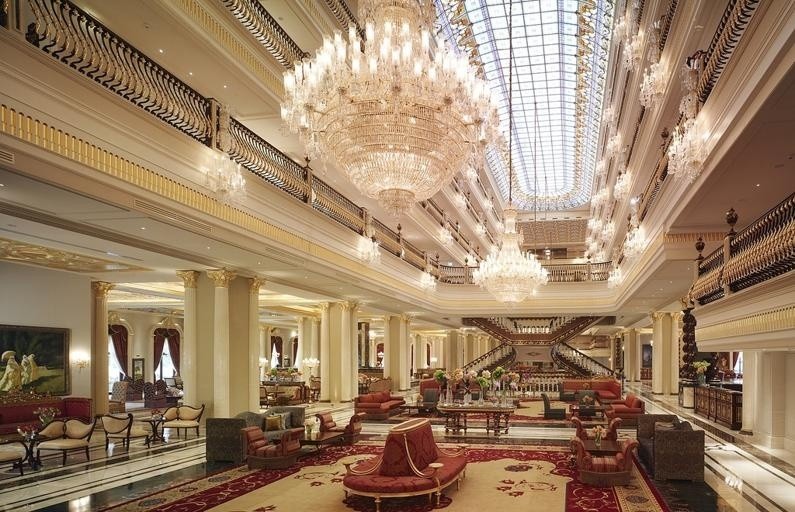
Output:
[0,324,71,396]
[132,358,145,382]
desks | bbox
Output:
[436,400,516,436]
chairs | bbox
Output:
[240,425,304,473]
[358,373,392,394]
[0,379,204,476]
[317,410,362,446]
[541,392,565,419]
[259,376,321,408]
[570,417,640,489]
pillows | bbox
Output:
[264,412,291,431]
[651,422,674,440]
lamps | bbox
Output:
[378,351,384,364]
[303,358,320,403]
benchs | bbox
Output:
[342,417,467,512]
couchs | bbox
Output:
[558,378,645,427]
[354,390,405,421]
[206,405,305,468]
[636,413,705,483]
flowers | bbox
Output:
[692,360,711,383]
[434,367,529,389]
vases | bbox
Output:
[699,374,706,386]
[436,393,513,409]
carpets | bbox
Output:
[389,398,608,429]
[95,440,670,512]
